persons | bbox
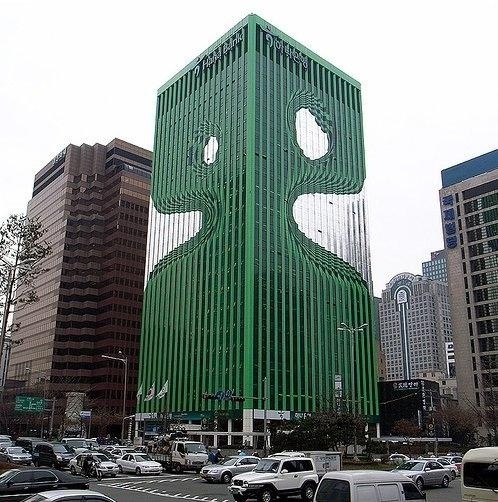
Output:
[83,454,97,478]
[214,449,260,463]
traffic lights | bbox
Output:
[203,392,217,400]
[231,396,246,402]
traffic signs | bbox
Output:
[14,395,46,413]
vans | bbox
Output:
[313,469,428,502]
[460,447,498,501]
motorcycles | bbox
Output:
[217,455,227,464]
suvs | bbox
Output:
[227,456,320,502]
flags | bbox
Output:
[145,381,155,401]
[156,380,168,400]
[135,383,142,400]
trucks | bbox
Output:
[267,450,344,479]
[149,440,209,474]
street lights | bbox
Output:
[100,350,128,446]
[336,322,369,464]
[25,366,47,438]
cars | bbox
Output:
[200,455,263,484]
[0,433,164,501]
[374,452,463,489]
